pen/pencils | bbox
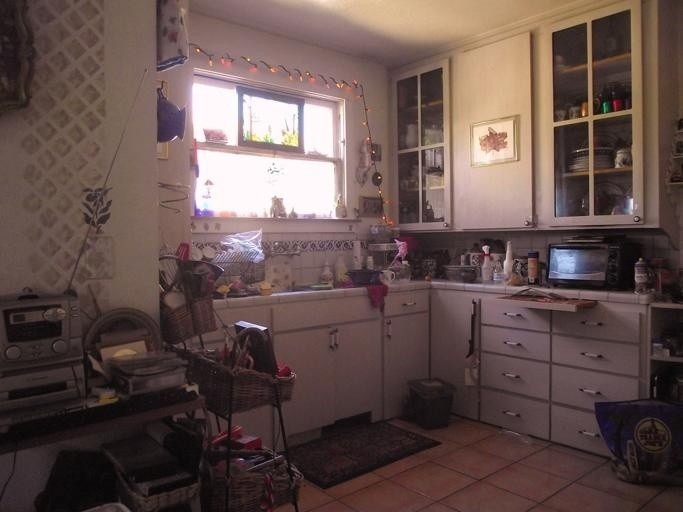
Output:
[215,333,250,368]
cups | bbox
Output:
[554,81,630,121]
[381,270,395,283]
[190,244,216,262]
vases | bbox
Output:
[404,123,419,150]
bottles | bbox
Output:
[503,240,514,280]
[335,194,346,217]
[526,250,539,286]
[334,254,347,284]
[399,260,410,281]
[350,239,361,270]
[364,256,373,269]
[460,253,464,266]
[632,258,650,294]
[492,259,503,283]
[318,255,333,283]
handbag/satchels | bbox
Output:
[594,400,680,484]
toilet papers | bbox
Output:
[191,246,215,262]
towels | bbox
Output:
[334,278,390,312]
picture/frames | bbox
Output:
[0,0,36,112]
[469,115,521,169]
[156,77,169,161]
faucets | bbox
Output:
[271,241,302,257]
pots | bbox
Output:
[345,268,383,285]
[660,330,682,356]
[441,263,479,282]
[664,377,682,402]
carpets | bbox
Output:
[279,420,442,492]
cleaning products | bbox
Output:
[367,256,375,270]
[320,256,334,285]
[335,253,350,281]
[481,245,492,281]
[352,240,362,270]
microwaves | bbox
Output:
[545,239,641,291]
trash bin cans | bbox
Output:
[407,377,456,430]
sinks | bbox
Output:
[272,286,314,293]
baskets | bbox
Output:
[166,345,295,415]
[159,295,217,344]
[100,427,303,512]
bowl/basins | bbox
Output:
[369,224,384,235]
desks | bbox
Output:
[0,378,206,511]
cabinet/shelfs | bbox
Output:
[207,294,272,452]
[648,298,682,413]
[451,17,536,235]
[536,0,675,238]
[431,281,478,423]
[271,290,382,452]
[666,115,681,189]
[549,287,647,461]
[384,279,430,419]
[478,282,548,443]
[385,53,450,239]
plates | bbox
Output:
[579,181,623,215]
[568,146,614,172]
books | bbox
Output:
[109,350,183,374]
